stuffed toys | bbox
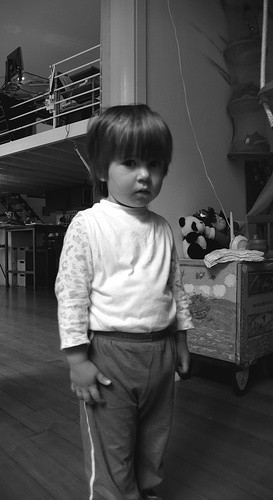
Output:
[179,209,241,259]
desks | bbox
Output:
[0,224,65,292]
[57,66,100,125]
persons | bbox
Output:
[53,105,194,500]
